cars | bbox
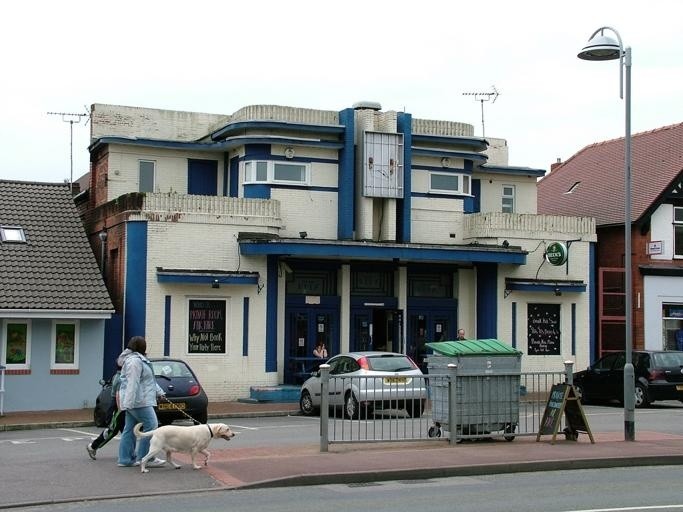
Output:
[94,356,208,434]
[299,350,428,421]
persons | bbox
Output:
[312,342,327,358]
[452,328,467,341]
[86,336,167,468]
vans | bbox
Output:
[573,348,683,409]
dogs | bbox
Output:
[132,422,236,474]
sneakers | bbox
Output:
[87,443,96,460]
[118,461,140,467]
[145,458,166,467]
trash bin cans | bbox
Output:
[422,340,524,442]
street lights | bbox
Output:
[577,26,636,442]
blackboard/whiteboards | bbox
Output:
[538,385,570,435]
